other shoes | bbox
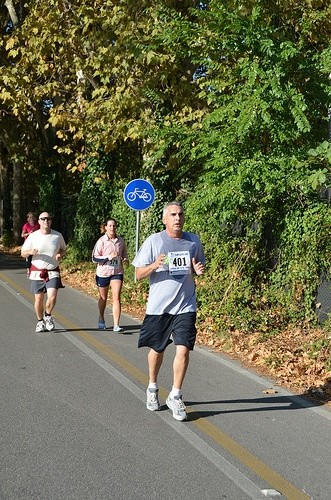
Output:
[98,320,106,331]
[113,327,124,332]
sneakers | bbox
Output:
[165,394,188,421]
[146,387,161,411]
[36,321,44,332]
[43,314,54,331]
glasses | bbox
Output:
[38,217,52,220]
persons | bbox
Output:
[131,202,206,421]
[21,212,40,276]
[21,211,67,332]
[92,218,130,331]
